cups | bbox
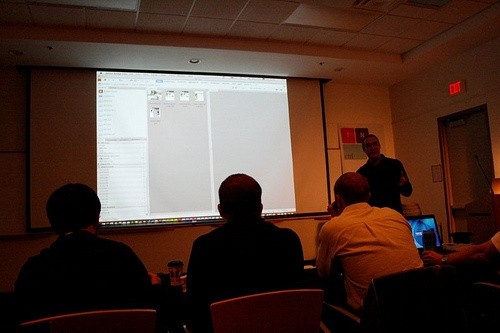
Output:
[168,260,183,281]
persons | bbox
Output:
[187,173,306,333]
[421,230,500,282]
[7,182,161,333]
[356,134,412,216]
[314,172,424,333]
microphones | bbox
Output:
[475,154,495,199]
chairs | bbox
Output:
[15,260,500,333]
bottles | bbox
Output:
[423,228,436,265]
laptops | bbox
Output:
[404,214,444,255]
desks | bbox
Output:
[155,259,343,333]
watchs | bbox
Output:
[440,255,448,265]
